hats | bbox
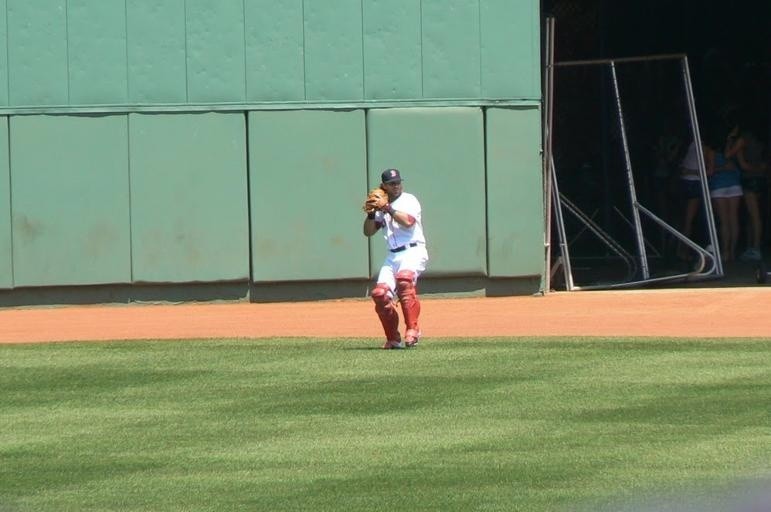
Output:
[381,169,403,184]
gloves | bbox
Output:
[363,193,392,214]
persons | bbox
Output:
[362,168,428,350]
[646,103,769,264]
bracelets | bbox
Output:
[387,207,396,216]
[367,212,376,219]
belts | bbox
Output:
[390,243,417,253]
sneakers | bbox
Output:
[738,249,763,261]
[383,340,401,348]
[405,336,417,347]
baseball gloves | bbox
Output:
[365,188,389,212]
